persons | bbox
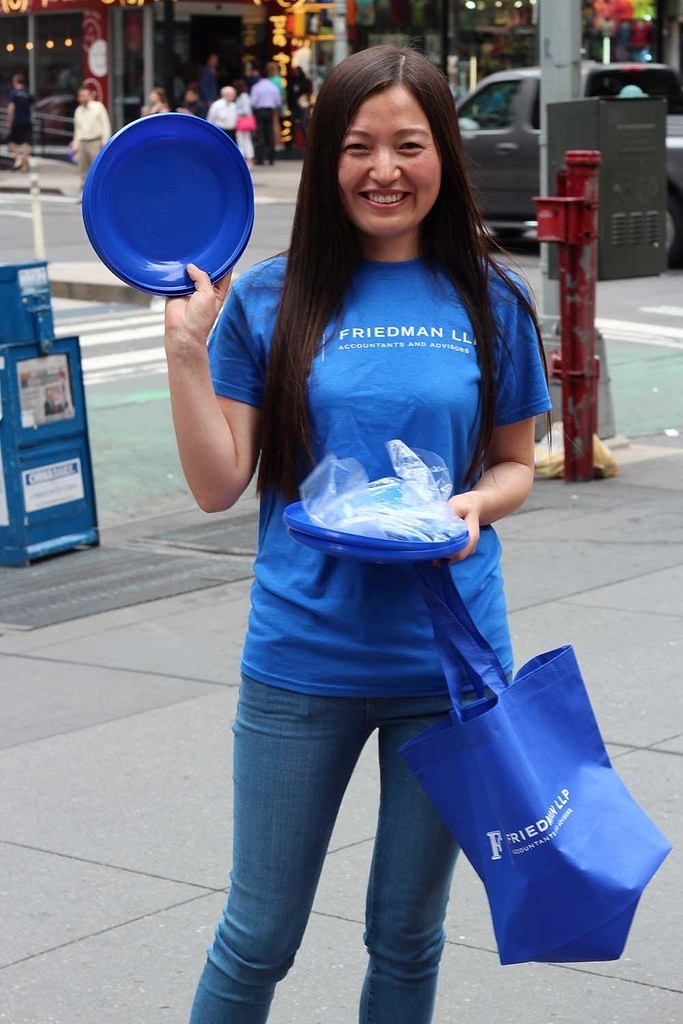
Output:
[6,75,35,173]
[68,85,112,205]
[595,14,659,64]
[135,51,314,178]
[160,41,554,1023]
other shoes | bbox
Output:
[12,157,27,171]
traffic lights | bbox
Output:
[285,12,321,35]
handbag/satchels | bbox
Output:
[236,113,257,132]
[401,554,674,965]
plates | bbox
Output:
[80,112,258,302]
[279,495,466,551]
[287,528,473,569]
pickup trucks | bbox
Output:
[456,64,683,270]
